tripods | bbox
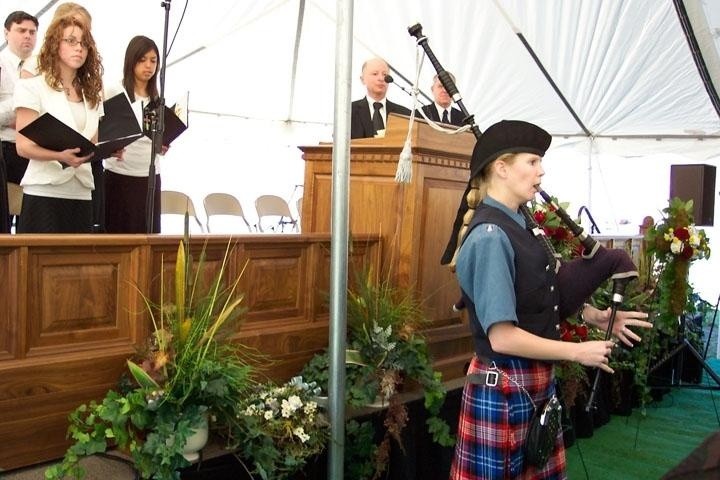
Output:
[644,261,719,390]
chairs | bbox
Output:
[297,196,302,213]
[6,183,29,224]
[156,191,205,233]
[202,193,250,230]
[256,194,291,231]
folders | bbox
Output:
[144,91,189,146]
[19,113,143,169]
[97,93,145,141]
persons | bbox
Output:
[439,121,654,480]
[53,1,100,34]
[413,71,473,129]
[351,57,412,139]
[1,8,38,234]
[14,15,102,233]
[98,34,190,232]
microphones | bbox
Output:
[384,75,433,122]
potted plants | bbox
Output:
[289,208,457,480]
[47,236,280,479]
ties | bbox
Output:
[441,109,450,125]
[371,102,385,138]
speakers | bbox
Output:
[670,165,716,226]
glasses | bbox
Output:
[60,36,92,49]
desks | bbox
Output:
[297,117,481,381]
[0,233,384,471]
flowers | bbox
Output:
[646,212,711,269]
[529,196,602,362]
[237,374,333,474]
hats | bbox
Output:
[440,119,552,267]
